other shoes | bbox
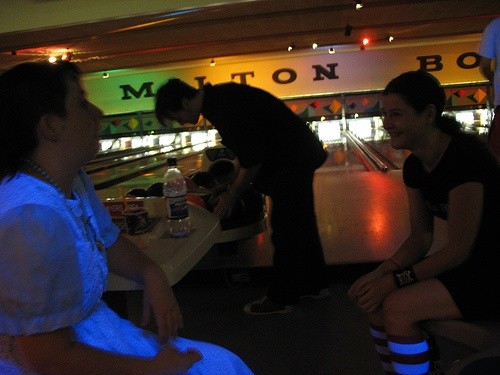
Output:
[243,296,289,315]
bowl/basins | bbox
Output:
[121,208,150,234]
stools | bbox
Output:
[410,310,500,374]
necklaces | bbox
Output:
[26,158,105,253]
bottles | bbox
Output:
[163,158,190,236]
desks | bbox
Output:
[94,196,224,292]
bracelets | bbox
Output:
[393,266,418,289]
[388,256,403,269]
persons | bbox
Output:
[478,18,500,163]
[156,78,329,316]
[347,70,500,375]
[0,62,253,375]
